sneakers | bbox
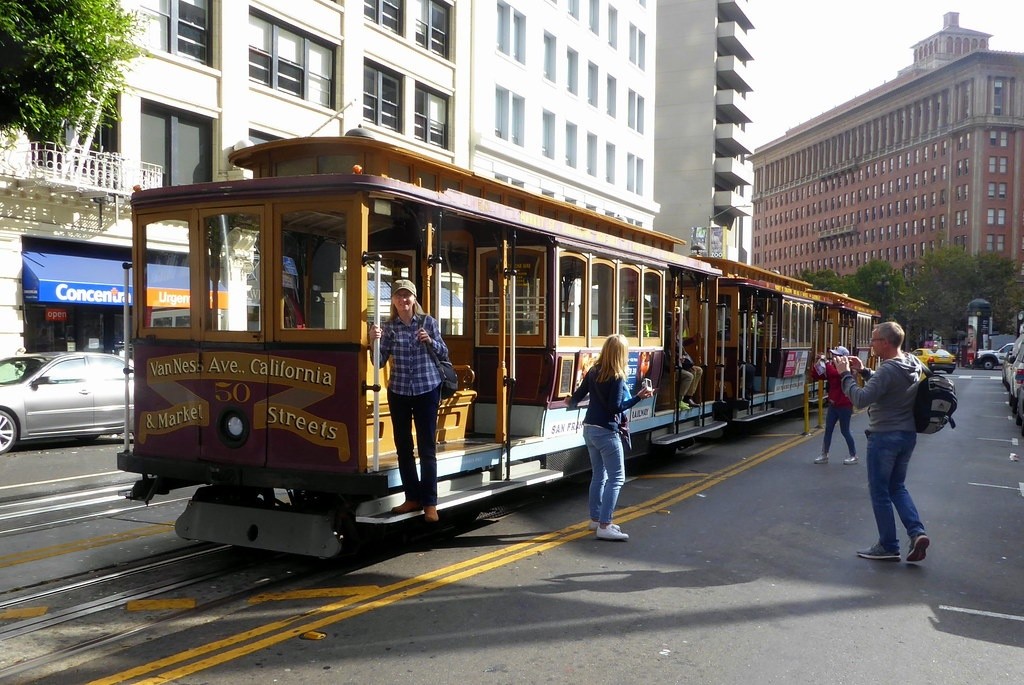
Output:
[589,521,620,531]
[597,526,629,539]
[856,541,900,560]
[906,534,930,561]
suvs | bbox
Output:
[974,344,1015,370]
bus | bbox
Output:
[113,122,882,560]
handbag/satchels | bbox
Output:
[618,413,632,451]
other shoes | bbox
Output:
[684,398,700,406]
[679,400,690,410]
[843,456,858,464]
[391,500,422,512]
[424,506,439,522]
[814,455,829,464]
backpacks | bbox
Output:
[912,358,957,434]
[390,317,458,399]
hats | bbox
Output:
[391,279,416,297]
[829,346,849,356]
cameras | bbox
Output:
[641,378,652,391]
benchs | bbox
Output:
[367,352,477,456]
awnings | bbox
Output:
[22,251,228,310]
[367,279,463,319]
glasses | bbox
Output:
[869,338,890,344]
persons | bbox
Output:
[809,346,858,465]
[833,322,930,561]
[665,318,703,410]
[564,333,650,540]
[968,325,977,361]
[369,279,450,521]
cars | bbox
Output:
[0,352,134,455]
[910,349,956,375]
[1002,333,1024,438]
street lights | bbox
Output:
[708,204,753,257]
